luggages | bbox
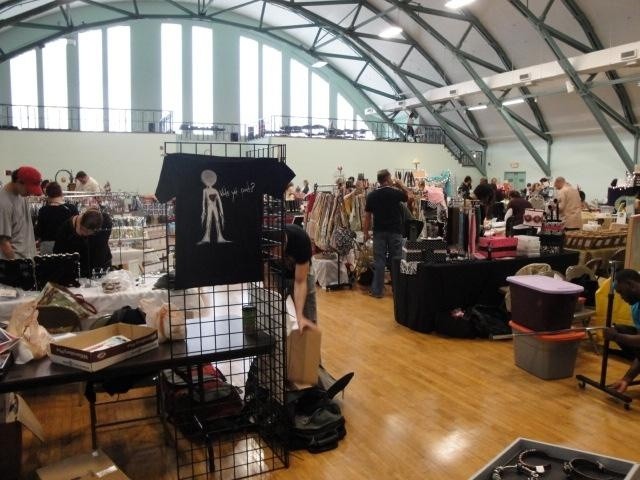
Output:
[156,362,244,442]
[245,353,355,453]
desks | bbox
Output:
[179,121,366,140]
[389,249,581,340]
[0,315,277,472]
[0,275,204,334]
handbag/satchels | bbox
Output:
[33,282,97,334]
[404,184,422,201]
[307,218,356,255]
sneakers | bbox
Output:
[368,289,383,298]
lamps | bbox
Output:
[378,2,404,39]
[468,96,525,112]
[311,60,328,68]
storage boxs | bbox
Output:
[285,329,321,385]
[506,274,586,380]
[36,449,131,480]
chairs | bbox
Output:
[566,265,608,310]
[510,263,599,354]
[0,252,81,291]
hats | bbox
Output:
[16,167,43,196]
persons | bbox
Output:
[404,112,417,142]
[264,223,317,335]
[302,179,309,194]
[603,269,640,393]
[0,166,112,278]
[363,169,414,297]
[457,175,583,230]
[287,183,296,201]
